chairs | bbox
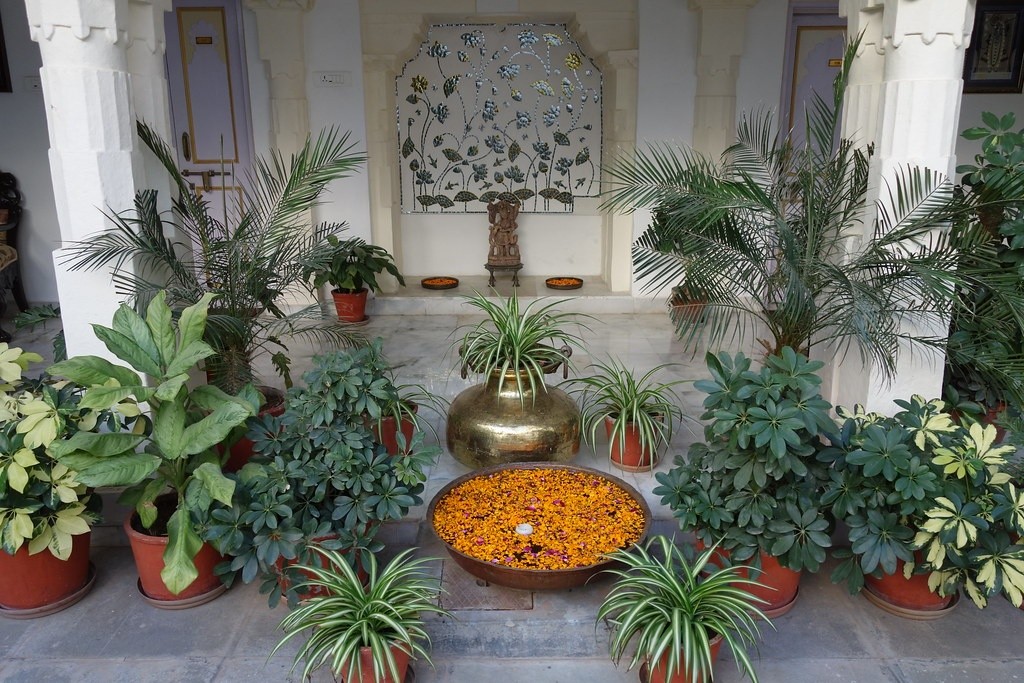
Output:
[0,171,31,343]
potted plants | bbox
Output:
[597,538,763,683]
[0,343,99,621]
[590,22,1023,386]
[113,107,373,465]
[937,111,1023,443]
[445,285,582,470]
[563,360,674,472]
[324,239,401,327]
[838,392,1024,620]
[671,260,714,327]
[352,338,436,458]
[249,418,371,602]
[69,290,240,610]
[279,552,444,683]
[653,345,830,619]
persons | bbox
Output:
[489,209,518,256]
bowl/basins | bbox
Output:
[426,461,652,591]
